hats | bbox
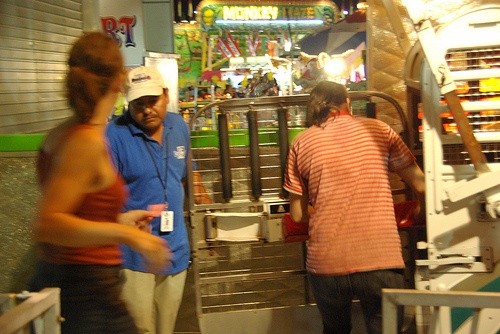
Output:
[126,66,166,102]
